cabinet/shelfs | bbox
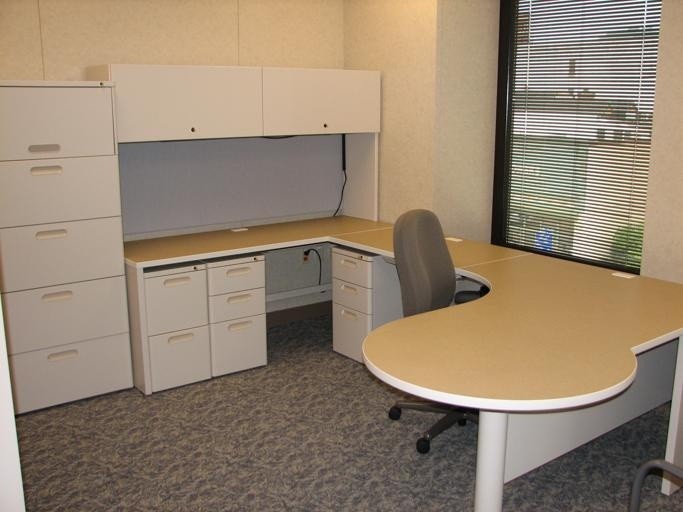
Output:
[0,81,134,420]
[143,249,271,397]
[324,244,397,373]
[85,61,380,148]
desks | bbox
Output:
[119,211,391,268]
[358,213,683,511]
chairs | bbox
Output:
[388,207,487,455]
